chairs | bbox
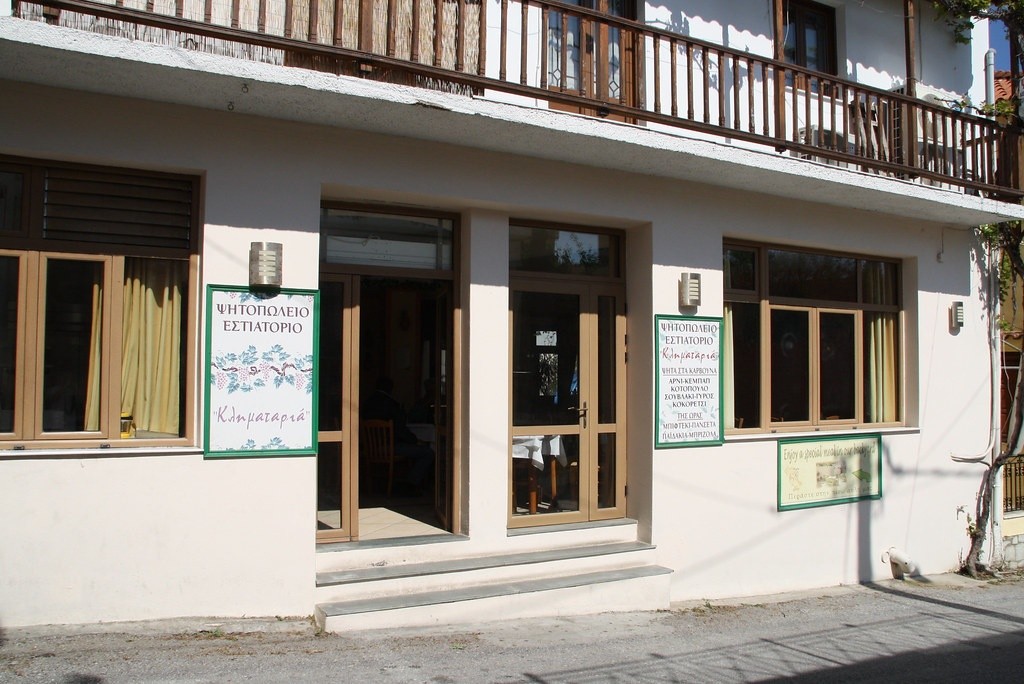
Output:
[359,418,415,500]
[321,416,347,489]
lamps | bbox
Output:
[250,241,283,287]
[952,301,965,327]
[680,272,701,306]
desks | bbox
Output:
[513,435,568,514]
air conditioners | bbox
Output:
[798,126,835,173]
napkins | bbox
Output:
[883,82,968,194]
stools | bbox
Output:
[570,462,600,499]
